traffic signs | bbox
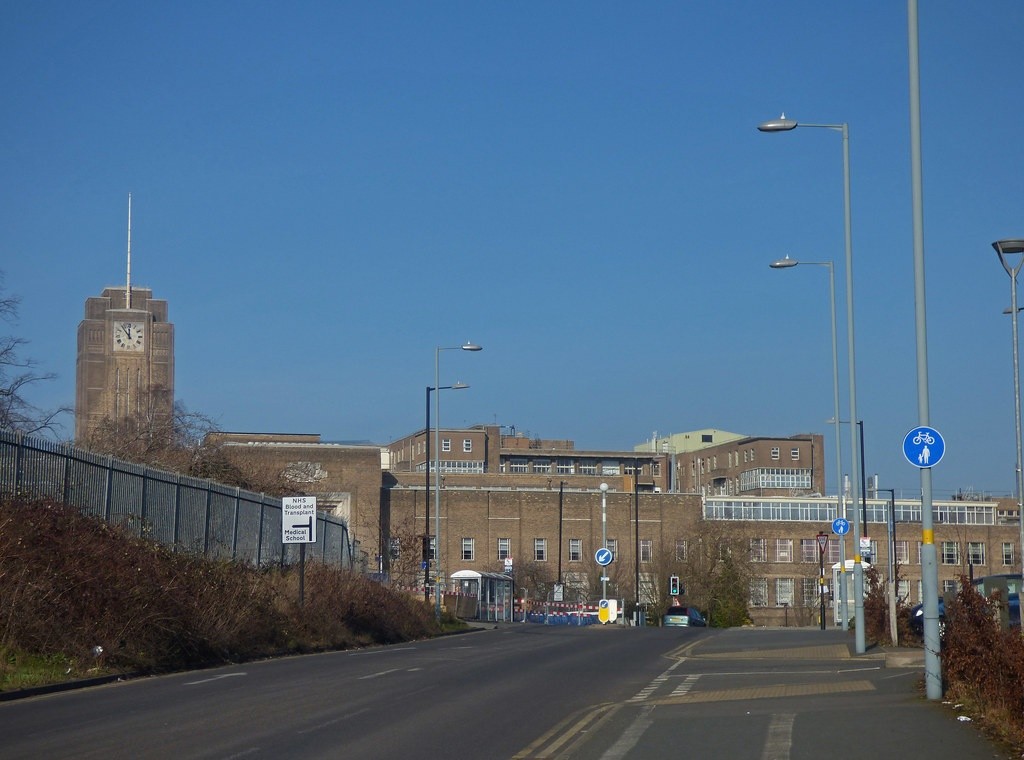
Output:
[282,496,317,545]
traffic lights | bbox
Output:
[668,574,684,597]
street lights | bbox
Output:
[759,118,867,657]
[769,257,851,633]
[826,418,872,565]
[432,341,483,619]
[422,383,470,606]
